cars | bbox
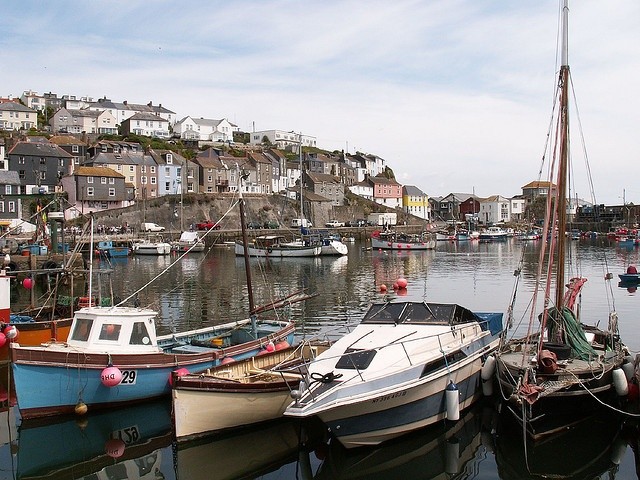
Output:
[196,219,222,230]
[245,221,264,229]
[264,220,279,229]
[141,222,166,232]
[346,221,358,227]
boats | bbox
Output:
[70,239,134,258]
[619,271,639,287]
[371,228,436,249]
[436,226,518,243]
[235,230,348,257]
[517,226,567,241]
[0,276,122,388]
[128,239,171,256]
[284,304,504,451]
[168,238,206,252]
[171,339,339,449]
[9,306,294,422]
[566,229,640,245]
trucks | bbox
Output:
[291,219,313,228]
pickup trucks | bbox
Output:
[326,219,345,227]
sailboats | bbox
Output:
[498,3,633,478]
